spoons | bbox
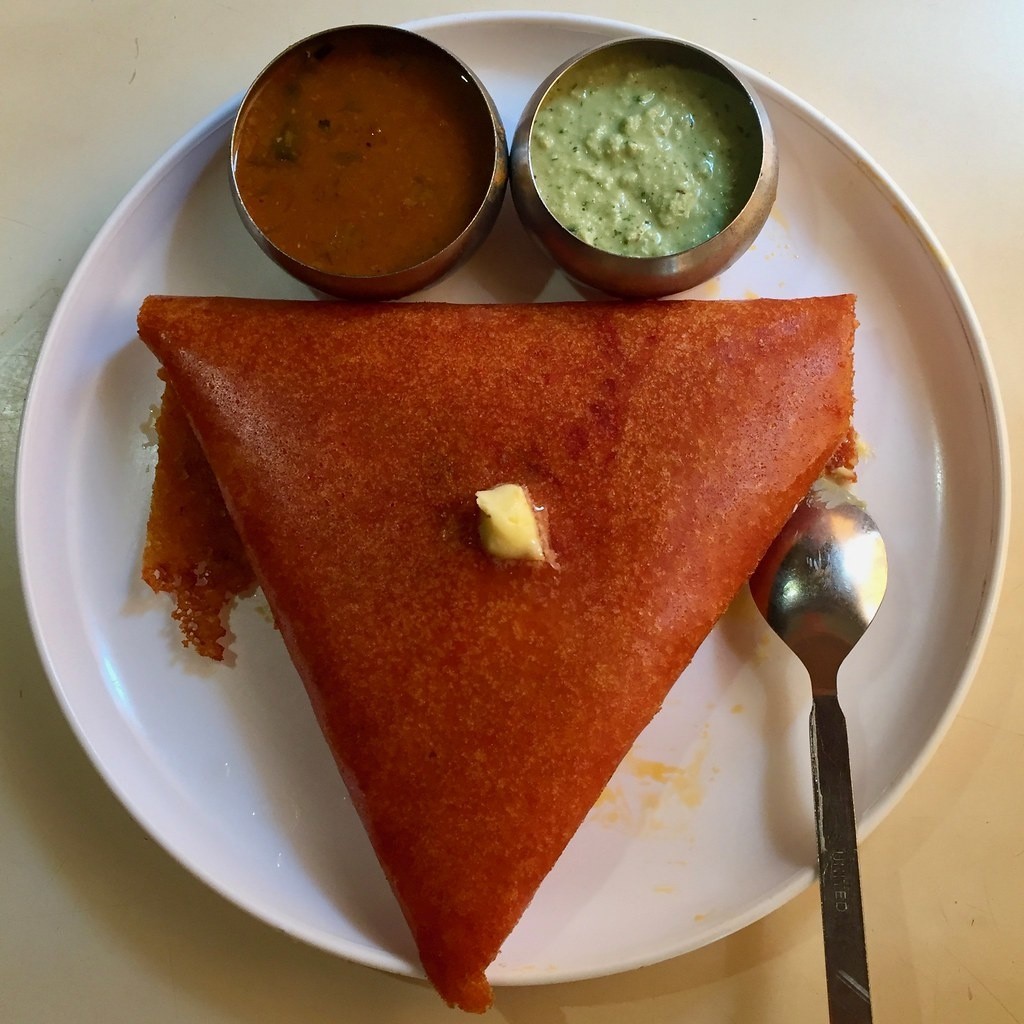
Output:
[750,493,890,1024]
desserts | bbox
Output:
[135,292,866,1015]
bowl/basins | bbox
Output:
[512,35,777,297]
[228,25,508,299]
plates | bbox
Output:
[14,13,1009,985]
